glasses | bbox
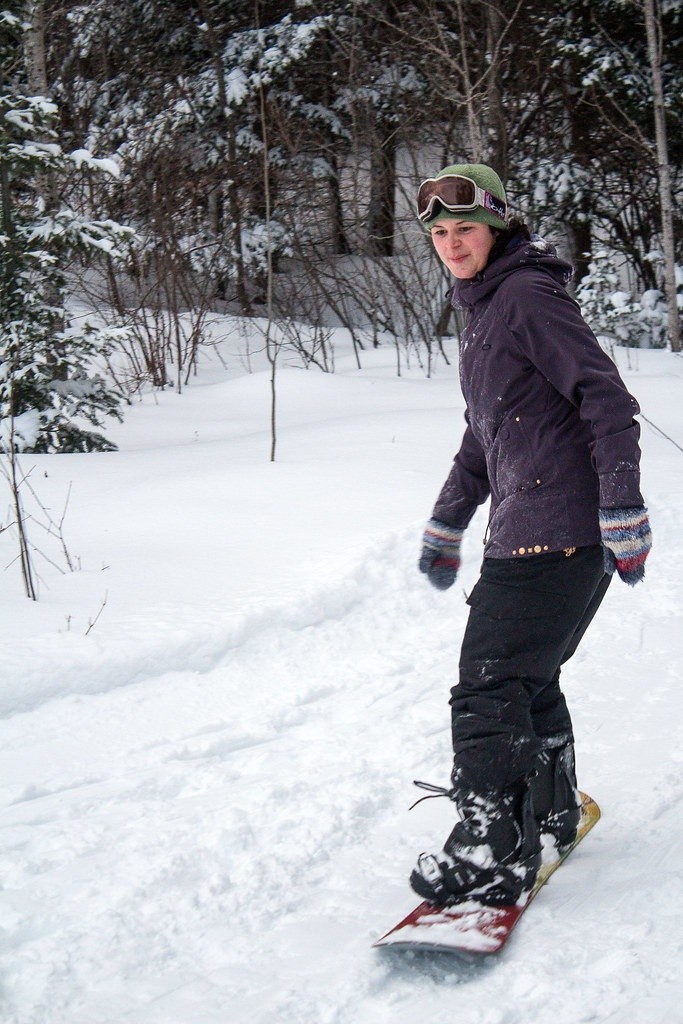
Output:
[416,174,481,221]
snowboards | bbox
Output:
[372,790,601,955]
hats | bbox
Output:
[426,163,509,231]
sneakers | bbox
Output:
[526,732,581,848]
[403,785,543,908]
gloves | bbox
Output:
[418,519,464,593]
[598,506,654,586]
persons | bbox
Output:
[410,165,651,905]
